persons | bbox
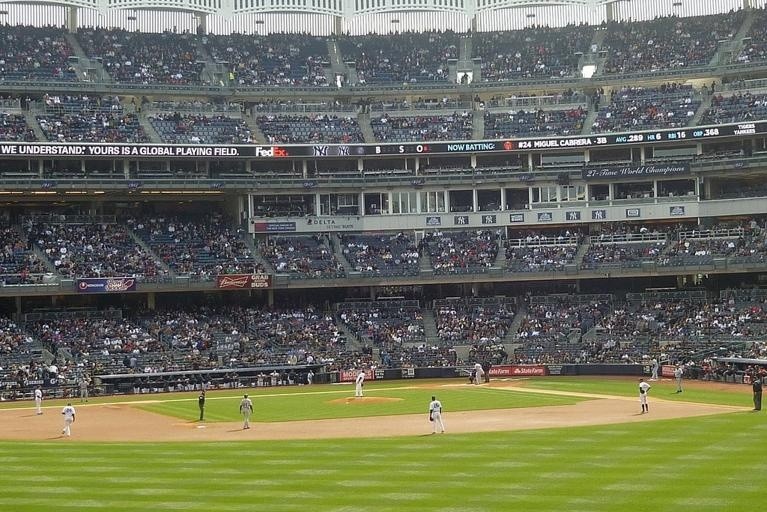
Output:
[240,394,254,429]
[0,6,767,401]
[430,396,445,434]
[639,378,651,414]
[199,391,205,421]
[35,385,42,415]
[753,376,762,410]
[61,402,75,437]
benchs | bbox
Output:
[1,18,766,399]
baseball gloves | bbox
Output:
[641,390,644,394]
[430,417,434,421]
